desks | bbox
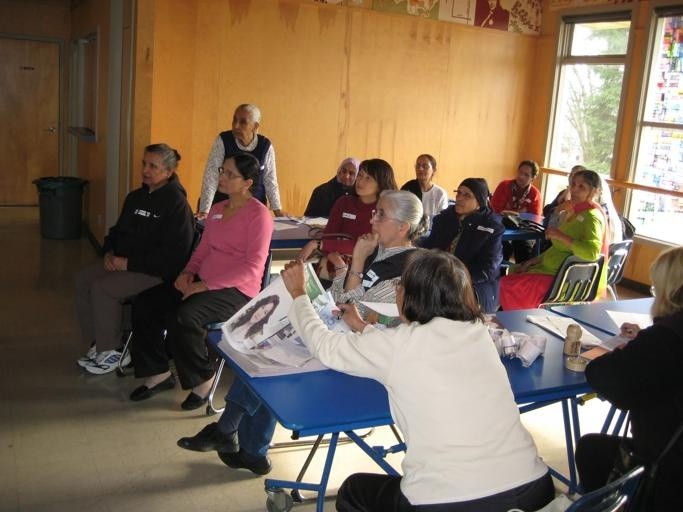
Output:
[205,292,682,512]
[192,206,551,291]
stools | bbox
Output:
[116,294,137,377]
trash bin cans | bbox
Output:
[32,176,87,240]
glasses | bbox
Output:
[218,167,246,180]
[650,285,657,298]
[395,278,405,295]
[371,208,405,224]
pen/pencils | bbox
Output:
[338,297,353,320]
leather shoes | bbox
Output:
[130,371,176,400]
[217,450,272,475]
[181,370,223,410]
[177,422,242,453]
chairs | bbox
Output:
[606,238,636,304]
[540,253,609,308]
[563,461,649,510]
[160,248,275,415]
[611,214,636,301]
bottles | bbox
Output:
[563,324,582,357]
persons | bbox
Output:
[130,153,274,410]
[575,248,683,512]
[196,104,288,221]
[76,144,195,374]
[281,244,554,512]
[178,190,432,474]
[226,295,279,345]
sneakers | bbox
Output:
[86,344,132,375]
[77,339,97,367]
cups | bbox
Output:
[493,332,547,366]
